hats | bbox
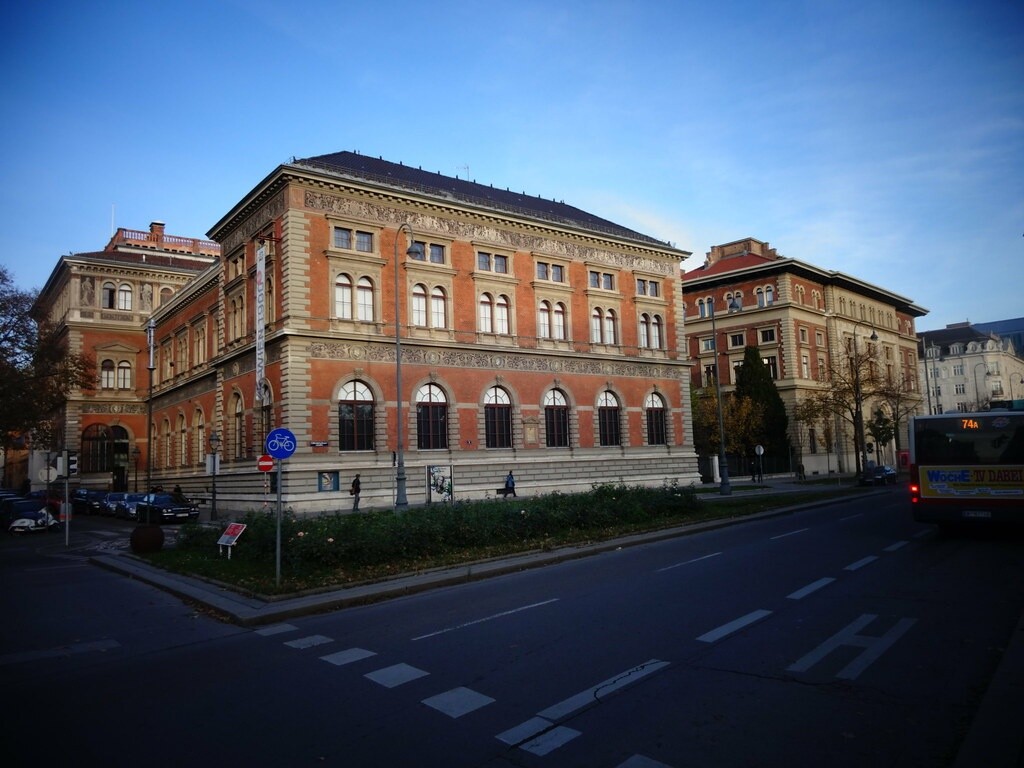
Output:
[176,485,179,486]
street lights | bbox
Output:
[393,222,425,513]
[711,283,741,497]
[853,320,879,481]
[1009,372,1024,410]
[209,430,223,521]
[974,362,992,413]
[924,346,946,415]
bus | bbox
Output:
[908,409,1024,534]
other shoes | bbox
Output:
[352,508,359,512]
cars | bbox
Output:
[0,491,60,536]
[73,490,201,525]
[873,466,897,487]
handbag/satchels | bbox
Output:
[349,487,356,495]
[505,481,509,488]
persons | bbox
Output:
[150,486,155,493]
[174,484,182,493]
[797,461,806,481]
[431,475,452,503]
[351,473,361,512]
[750,462,761,483]
[503,471,517,498]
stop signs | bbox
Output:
[259,456,274,471]
